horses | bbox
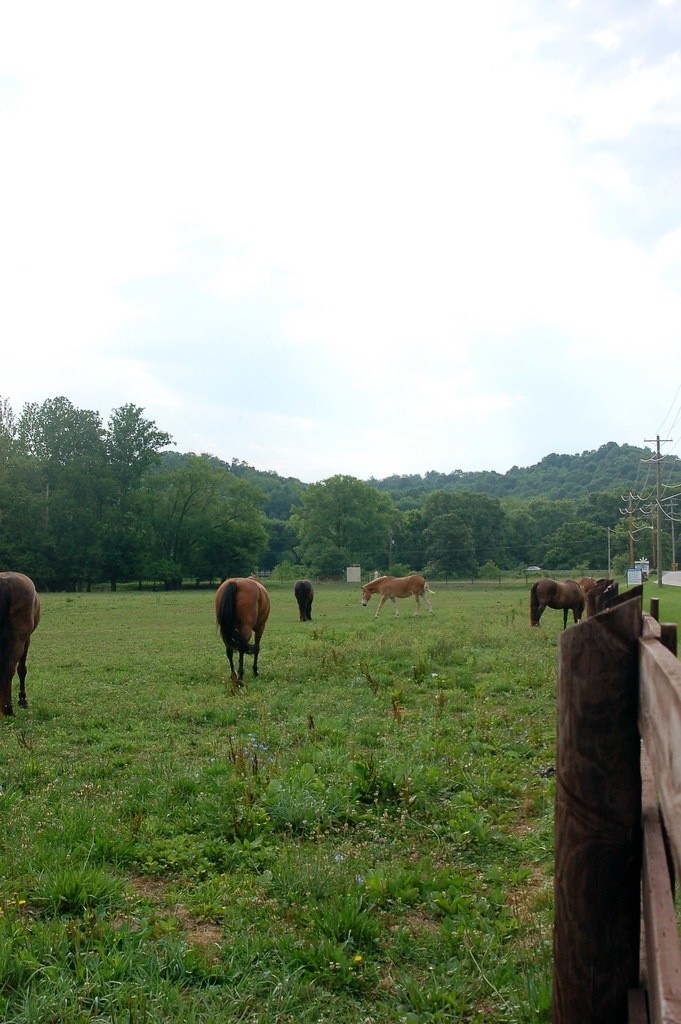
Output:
[360,574,437,619]
[295,580,314,622]
[530,577,614,633]
[0,570,40,716]
[215,576,271,689]
